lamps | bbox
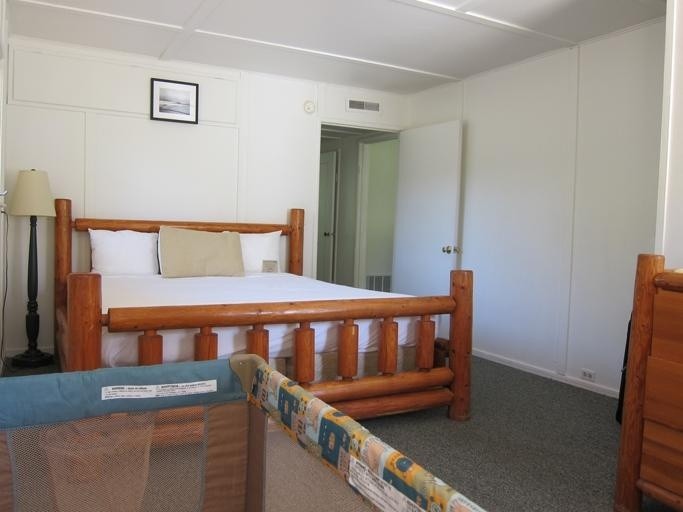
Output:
[8,169,57,369]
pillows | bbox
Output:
[159,225,245,278]
[240,229,283,276]
[87,228,160,276]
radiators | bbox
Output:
[367,272,392,292]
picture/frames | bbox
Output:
[150,78,199,124]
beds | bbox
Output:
[614,253,683,510]
[0,353,489,512]
[55,198,474,420]
